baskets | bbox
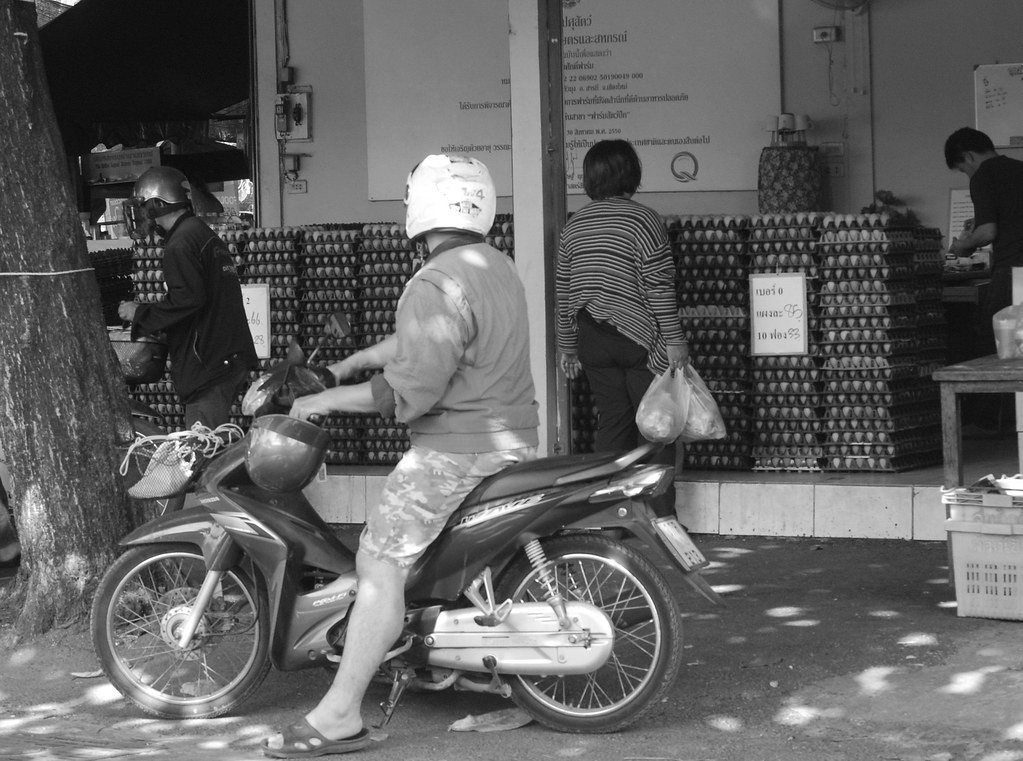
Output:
[110,340,168,384]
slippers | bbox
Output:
[260,718,369,759]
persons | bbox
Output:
[266,152,542,755]
[554,138,693,550]
[943,126,1021,450]
[116,165,265,451]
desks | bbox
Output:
[932,351,1023,490]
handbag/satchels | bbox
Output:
[637,363,727,442]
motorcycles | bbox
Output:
[89,311,726,741]
[110,339,188,519]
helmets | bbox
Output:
[132,166,191,208]
[246,413,331,495]
[406,154,496,243]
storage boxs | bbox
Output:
[81,143,163,182]
[942,488,1022,623]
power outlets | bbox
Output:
[813,26,838,43]
